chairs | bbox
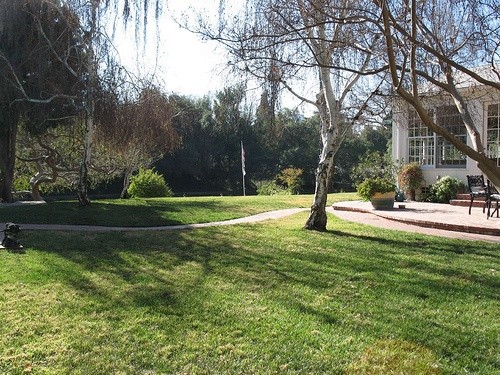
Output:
[486,179,500,220]
[466,174,489,215]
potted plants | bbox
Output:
[352,150,396,208]
[400,162,464,204]
